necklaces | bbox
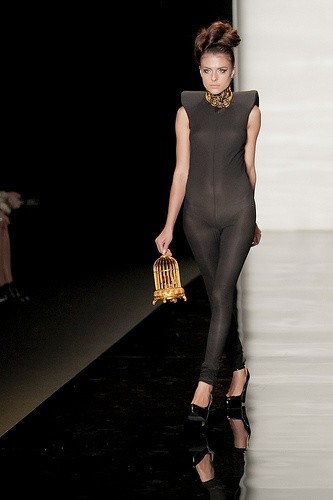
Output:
[204,86,234,108]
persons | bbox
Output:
[0,189,41,280]
[154,19,262,424]
[184,410,252,500]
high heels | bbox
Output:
[223,369,250,410]
[188,392,212,426]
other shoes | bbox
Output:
[0,281,30,304]
